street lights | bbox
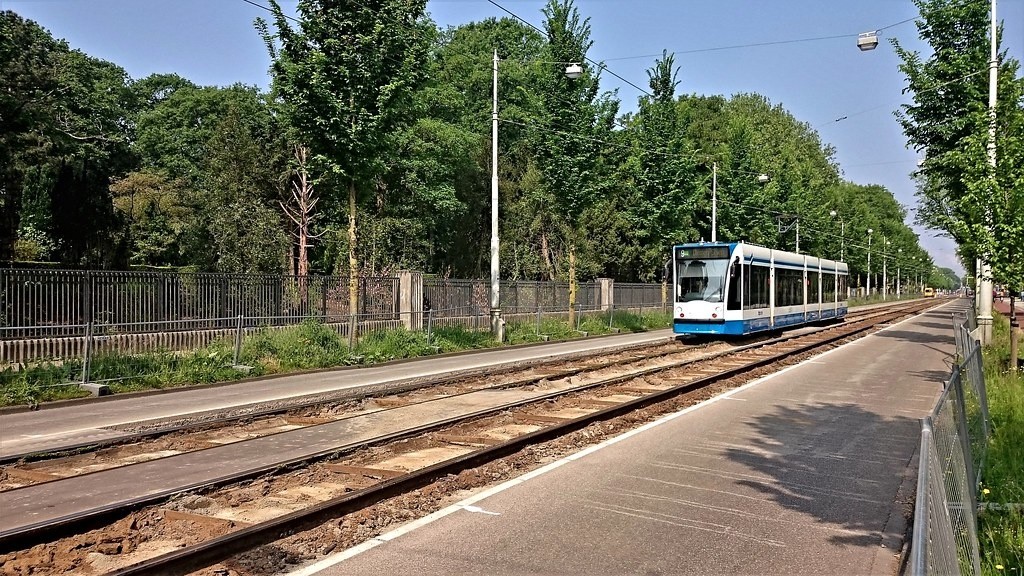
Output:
[840,222,931,301]
[857,1,1000,354]
[711,161,770,242]
[490,48,584,340]
[796,205,838,252]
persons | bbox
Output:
[1020,290,1024,301]
[1000,290,1004,301]
[993,291,997,302]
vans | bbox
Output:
[924,288,935,298]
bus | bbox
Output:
[663,243,849,338]
